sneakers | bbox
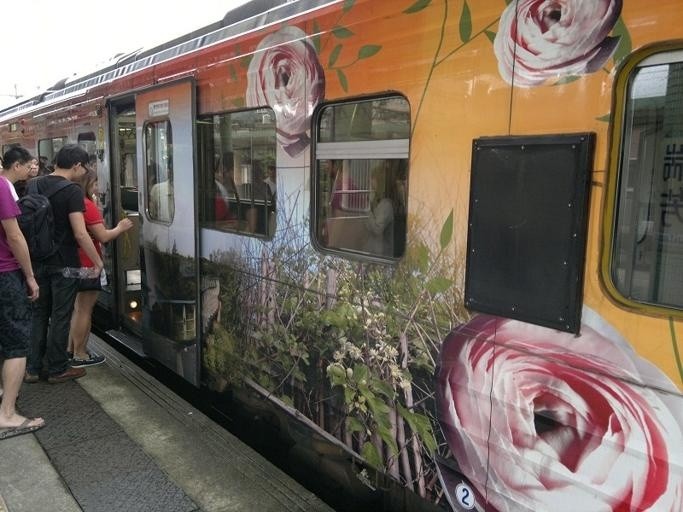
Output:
[23,369,39,383]
[48,348,105,382]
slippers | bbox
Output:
[0,416,46,441]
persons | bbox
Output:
[0,141,133,384]
[0,174,46,440]
[148,156,174,223]
[211,152,409,254]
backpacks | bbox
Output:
[14,176,82,261]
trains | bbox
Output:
[0,0,683,512]
